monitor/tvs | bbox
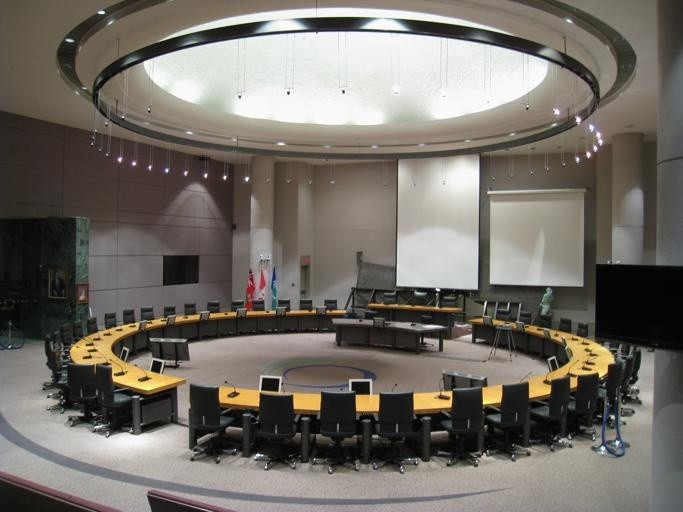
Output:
[150,358,166,374]
[120,346,130,362]
[562,336,566,346]
[565,344,572,360]
[547,356,559,372]
[542,328,551,338]
[259,375,282,392]
[199,311,210,321]
[483,316,493,326]
[373,317,386,328]
[516,322,525,331]
[276,307,286,316]
[349,379,373,395]
[236,308,247,317]
[138,320,147,331]
[166,315,176,326]
[316,306,327,316]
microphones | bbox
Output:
[340,387,344,392]
[391,383,397,392]
[85,334,98,352]
[109,359,125,376]
[566,359,579,377]
[224,381,239,398]
[96,352,112,365]
[520,371,531,383]
[281,383,286,395]
[134,364,147,381]
[434,378,450,400]
[76,346,93,358]
[82,337,94,346]
[543,369,556,385]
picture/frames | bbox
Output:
[46,264,70,300]
[76,283,89,304]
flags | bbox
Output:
[257,268,267,300]
[271,266,278,310]
[244,267,256,309]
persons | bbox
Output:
[78,287,87,300]
[539,287,554,317]
[50,270,65,297]
